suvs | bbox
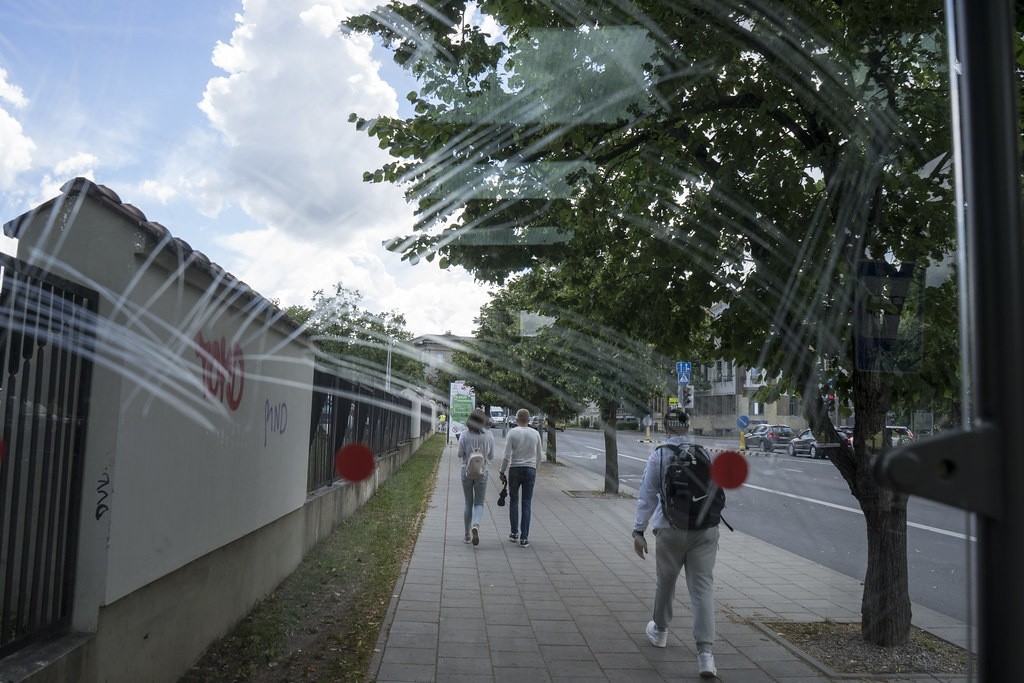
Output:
[744,423,794,452]
[850,426,914,453]
[542,419,566,432]
[788,429,848,459]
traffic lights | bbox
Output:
[682,386,691,406]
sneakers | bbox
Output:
[698,653,717,677]
[646,621,667,647]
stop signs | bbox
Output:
[828,392,835,399]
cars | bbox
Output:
[509,416,517,427]
[532,416,538,429]
[839,426,855,437]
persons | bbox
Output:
[437,412,447,431]
[456,409,495,547]
[500,408,542,547]
[633,409,725,677]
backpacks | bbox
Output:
[656,443,725,531]
[466,430,486,479]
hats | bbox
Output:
[663,410,689,432]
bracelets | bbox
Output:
[633,529,645,537]
[500,471,504,475]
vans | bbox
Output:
[483,406,508,428]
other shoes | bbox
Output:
[464,535,472,543]
[520,540,529,547]
[509,534,518,541]
[472,527,479,546]
